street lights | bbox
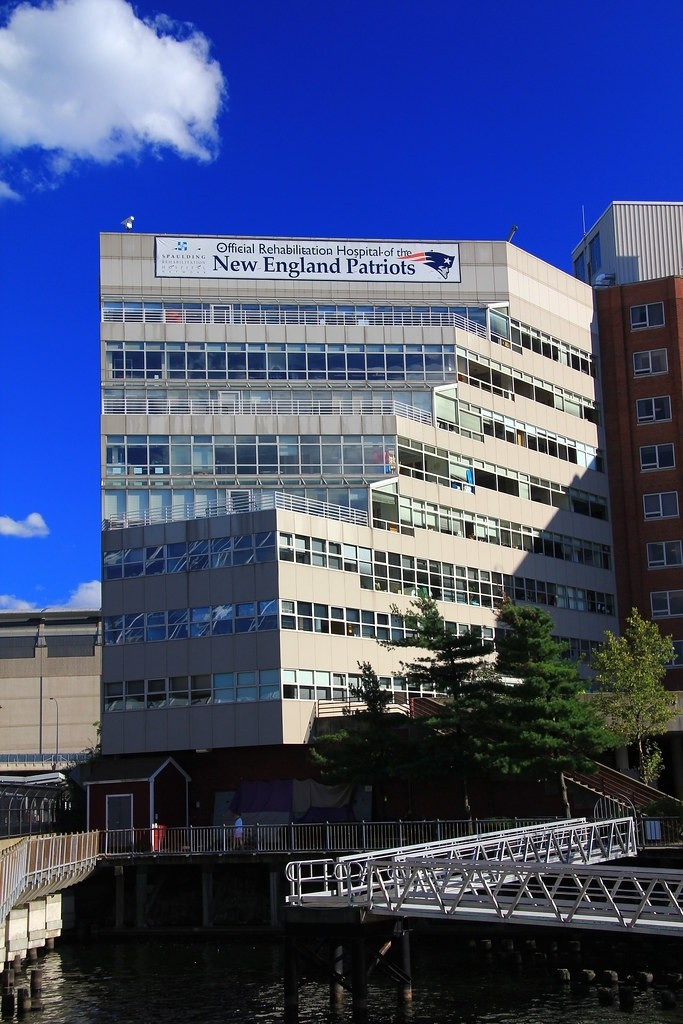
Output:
[50,697,59,764]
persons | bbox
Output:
[233,812,244,850]
